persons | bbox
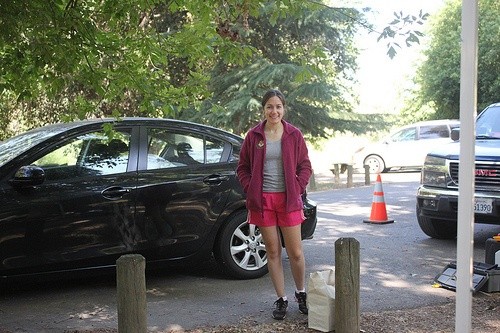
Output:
[237,88,314,321]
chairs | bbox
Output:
[92,139,192,166]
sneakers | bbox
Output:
[294,290,308,315]
[272,296,288,320]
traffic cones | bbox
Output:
[363,174,394,224]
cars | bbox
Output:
[1,115,318,290]
[354,118,464,173]
[416,103,500,238]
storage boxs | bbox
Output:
[433,261,500,293]
[307,269,336,332]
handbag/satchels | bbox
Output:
[308,269,335,332]
